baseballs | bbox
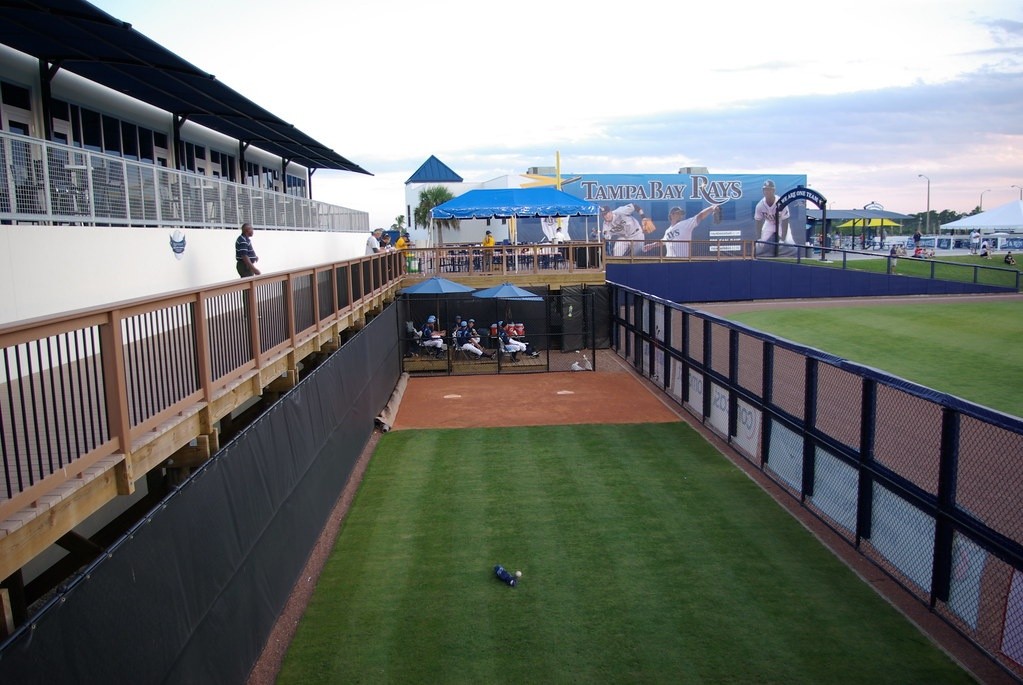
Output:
[515,571,522,578]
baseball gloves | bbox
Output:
[713,206,722,224]
[641,218,656,234]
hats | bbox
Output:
[599,205,609,214]
[427,316,436,322]
[456,315,461,319]
[762,180,775,189]
[461,321,467,326]
[670,206,685,214]
[469,319,474,322]
[498,321,503,326]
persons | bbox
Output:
[500,322,539,357]
[755,181,796,248]
[236,223,261,319]
[600,203,656,256]
[418,316,444,358]
[394,235,410,249]
[833,229,887,250]
[970,228,992,259]
[1004,251,1016,265]
[365,229,394,255]
[452,316,496,359]
[556,227,564,245]
[913,229,923,248]
[591,228,612,255]
[642,205,720,257]
[890,243,934,259]
[483,230,494,271]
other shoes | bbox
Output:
[491,351,496,359]
[436,355,446,359]
[510,358,520,362]
[532,351,541,356]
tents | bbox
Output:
[429,186,602,272]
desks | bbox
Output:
[64,164,93,214]
[190,186,213,223]
[425,252,562,270]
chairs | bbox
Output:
[412,326,526,365]
[7,160,319,231]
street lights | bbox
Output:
[1010,184,1023,200]
[919,174,930,237]
[979,189,992,213]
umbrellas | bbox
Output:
[399,277,476,330]
[472,281,543,319]
[838,219,903,226]
